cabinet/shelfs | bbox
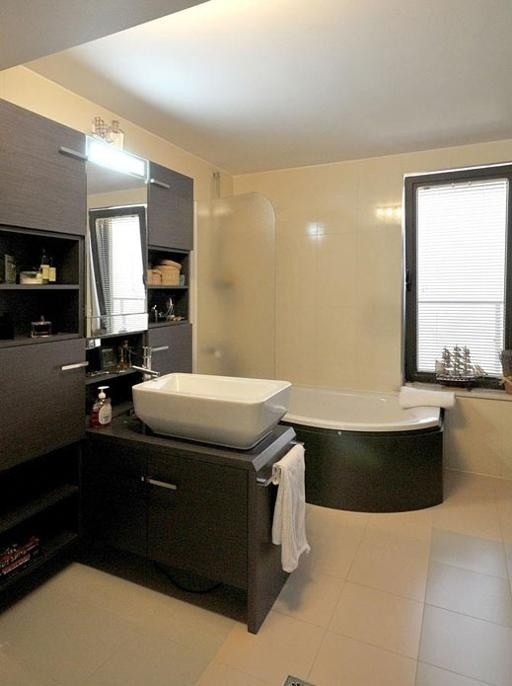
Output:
[0,98,85,237]
[1,225,83,340]
[3,442,86,611]
[86,438,248,591]
[1,339,88,470]
[146,161,195,387]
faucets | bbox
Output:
[132,346,170,379]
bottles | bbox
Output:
[41,248,57,284]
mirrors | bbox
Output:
[82,137,151,340]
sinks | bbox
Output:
[131,373,292,442]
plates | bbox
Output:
[159,260,182,271]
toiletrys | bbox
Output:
[91,385,113,429]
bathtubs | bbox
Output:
[282,385,441,432]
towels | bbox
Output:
[398,385,457,411]
[271,444,312,574]
